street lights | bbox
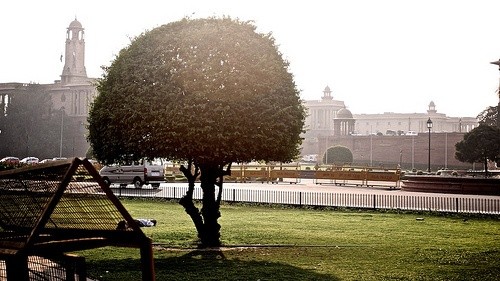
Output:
[427,117,433,172]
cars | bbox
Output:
[1,156,67,164]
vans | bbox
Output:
[99,154,167,189]
